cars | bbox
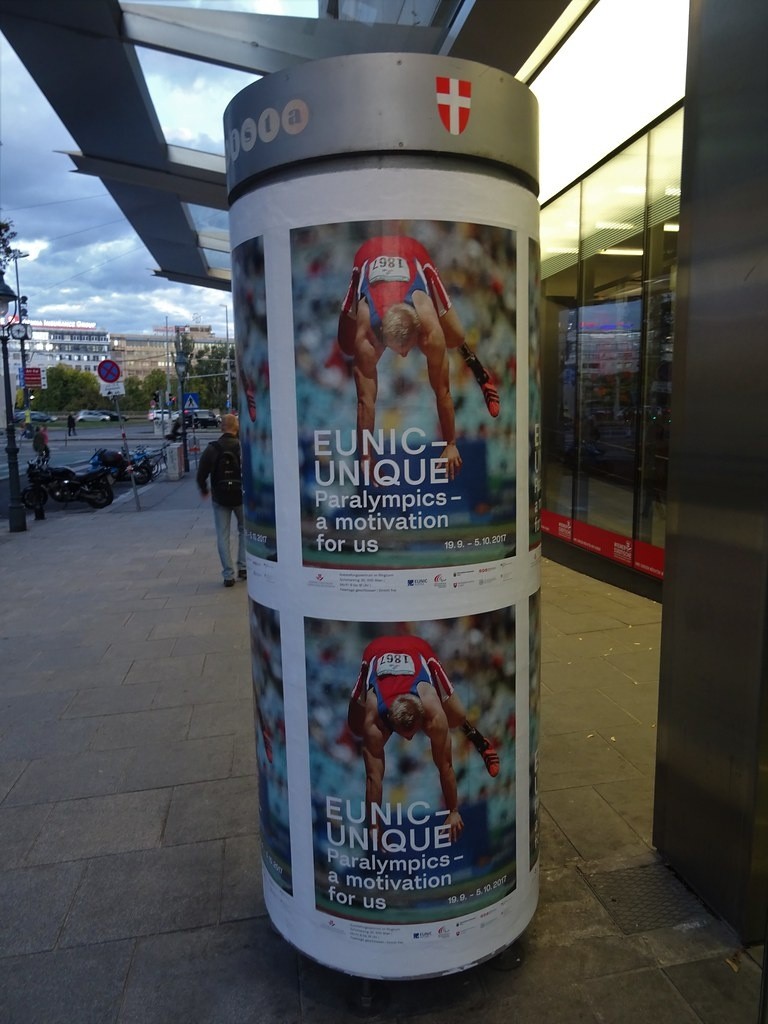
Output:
[100,409,129,422]
[174,408,240,431]
[148,409,180,422]
[16,409,57,424]
[73,409,112,423]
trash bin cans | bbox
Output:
[166,443,185,480]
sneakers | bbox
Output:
[476,369,500,417]
[478,738,500,777]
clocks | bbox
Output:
[12,324,33,339]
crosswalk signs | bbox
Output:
[182,393,200,411]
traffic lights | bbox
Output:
[153,386,161,402]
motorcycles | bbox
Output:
[21,441,124,510]
[87,440,172,485]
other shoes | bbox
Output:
[224,578,235,587]
[239,570,247,579]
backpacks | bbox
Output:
[207,440,243,506]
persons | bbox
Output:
[253,679,273,765]
[171,413,188,440]
[33,426,50,465]
[337,236,500,487]
[336,636,500,853]
[235,336,256,422]
[196,413,248,587]
[67,411,77,436]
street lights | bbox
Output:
[220,304,231,413]
[0,269,28,534]
[175,352,190,472]
